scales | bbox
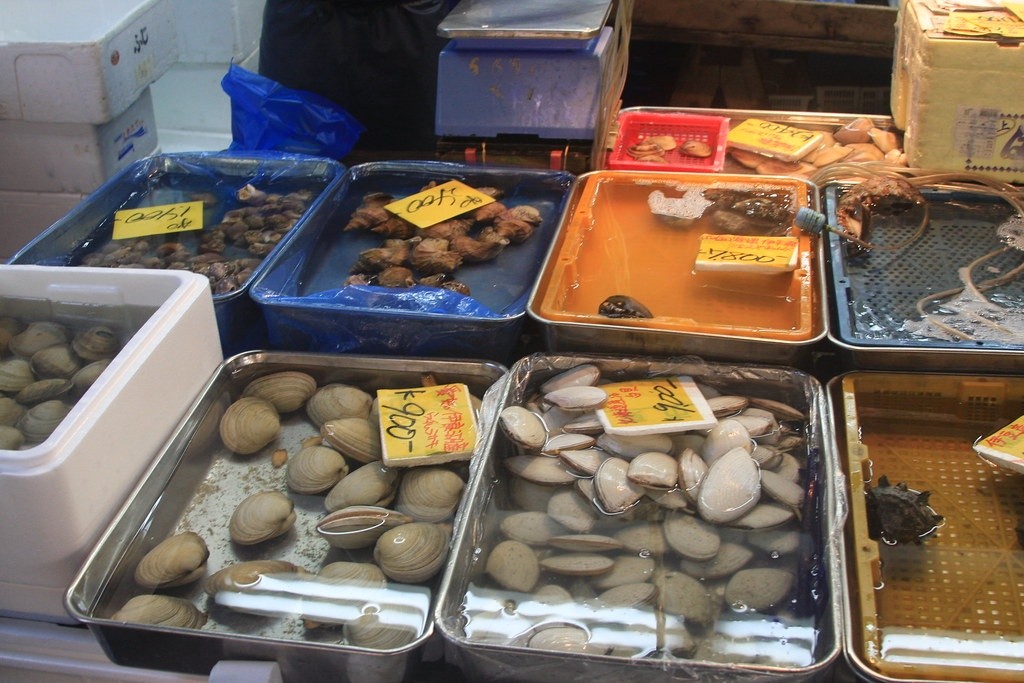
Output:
[434,0,617,139]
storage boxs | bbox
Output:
[149,48,260,134]
[5,150,349,358]
[156,128,232,153]
[0,0,179,126]
[0,263,224,620]
[667,43,891,114]
[0,144,162,258]
[63,161,1024,683]
[174,0,267,64]
[7,84,159,195]
[890,0,1024,184]
